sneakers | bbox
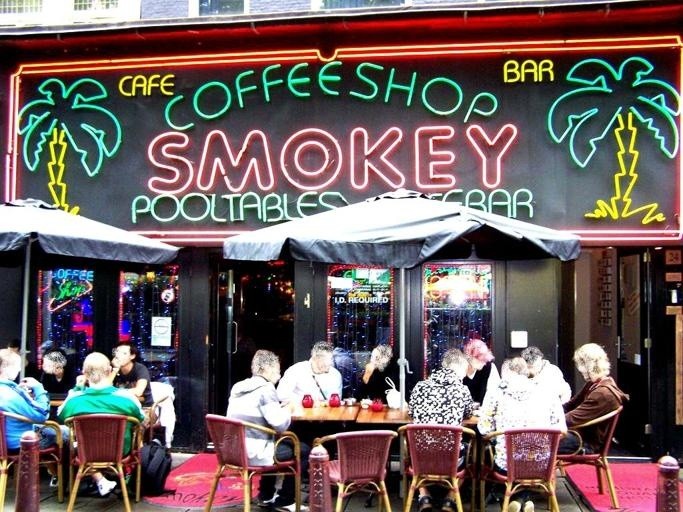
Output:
[508,501,521,512]
[49,474,58,488]
[275,503,310,512]
[96,477,117,497]
[257,492,279,507]
[522,501,534,512]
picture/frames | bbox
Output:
[419,258,496,380]
[327,264,395,354]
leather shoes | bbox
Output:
[417,495,433,512]
[441,497,457,512]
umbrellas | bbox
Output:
[222,186,580,499]
[0,198,184,381]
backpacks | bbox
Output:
[128,439,173,496]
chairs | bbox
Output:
[313,429,398,512]
[64,413,142,512]
[398,423,478,512]
[555,404,625,509]
[480,428,568,512]
[203,413,301,512]
[142,380,169,442]
[0,410,65,512]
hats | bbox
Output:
[463,339,495,363]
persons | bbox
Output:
[332,334,361,395]
[111,342,158,447]
[57,352,144,484]
[276,342,343,460]
[220,349,311,507]
[355,345,411,403]
[408,348,474,512]
[554,342,631,455]
[0,344,117,497]
[462,338,500,409]
[520,346,572,405]
[478,357,568,511]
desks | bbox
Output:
[50,399,157,430]
[282,402,482,506]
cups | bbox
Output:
[371,398,384,412]
[301,394,313,408]
[329,394,340,407]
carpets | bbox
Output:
[564,457,683,512]
[142,453,261,510]
